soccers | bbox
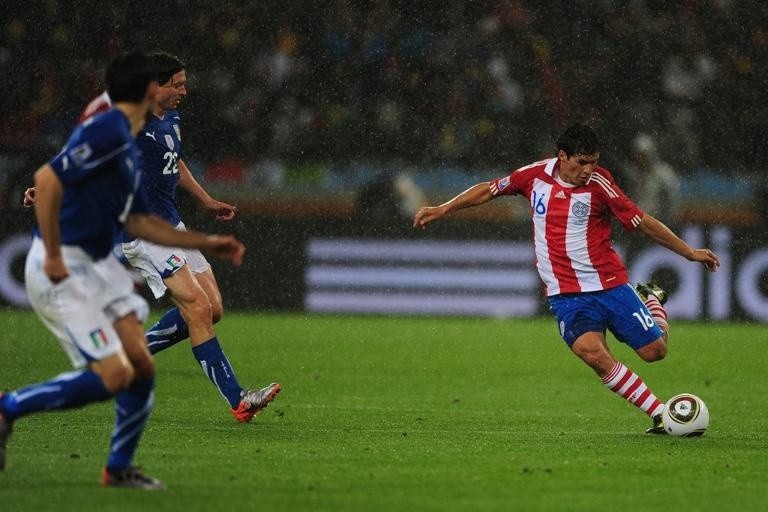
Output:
[662,393,709,438]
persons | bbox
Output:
[412,124,720,435]
[0,1,767,236]
[110,52,283,424]
[0,54,249,492]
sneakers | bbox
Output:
[101,463,162,490]
[645,413,667,434]
[228,380,281,423]
[0,390,13,472]
[634,280,668,305]
